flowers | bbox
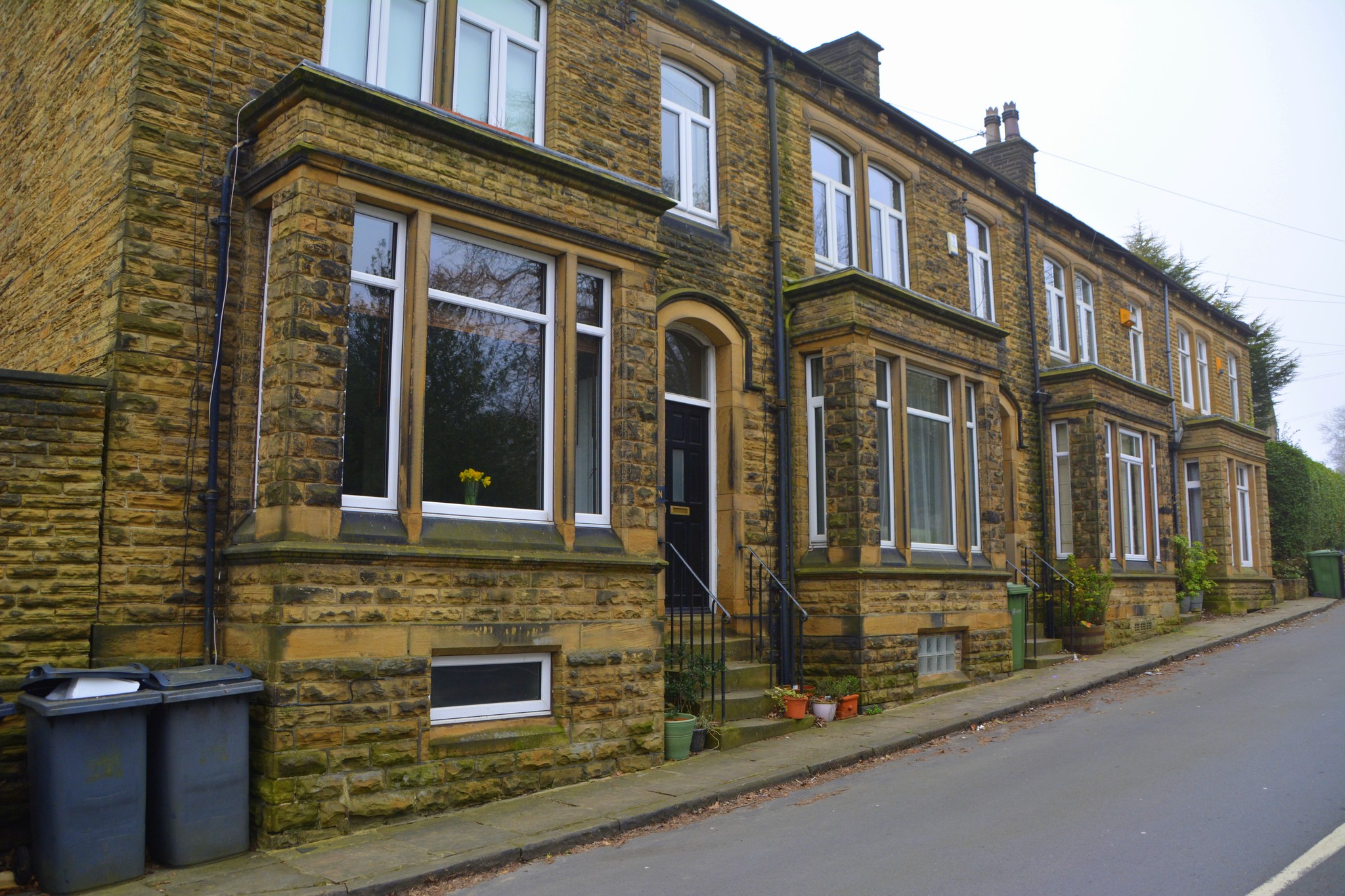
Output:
[459,468,491,488]
[765,686,806,713]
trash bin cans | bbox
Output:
[1302,549,1344,598]
[14,664,163,896]
[1007,582,1032,670]
[0,697,18,725]
[141,662,264,869]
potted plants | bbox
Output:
[1174,534,1218,614]
[811,675,860,722]
[664,630,729,760]
[1037,555,1116,655]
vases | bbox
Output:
[464,478,479,505]
[778,685,816,718]
